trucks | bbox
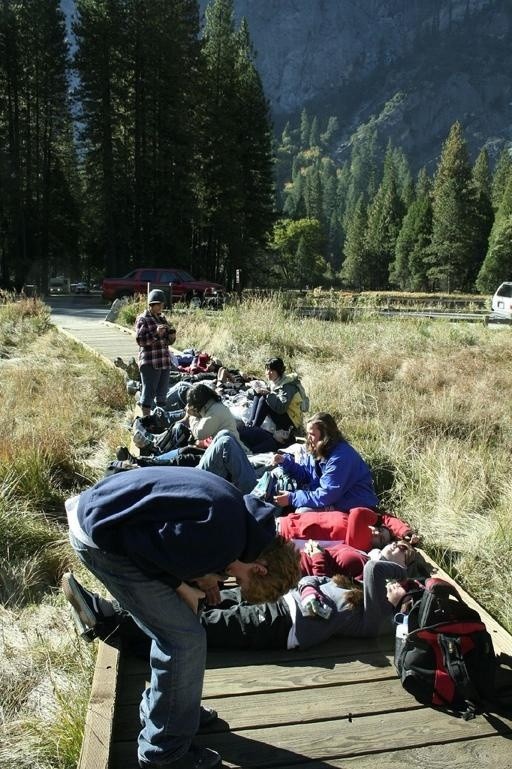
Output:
[48,277,65,294]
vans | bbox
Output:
[491,281,512,321]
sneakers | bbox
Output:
[61,573,98,629]
[193,703,215,732]
[166,748,222,769]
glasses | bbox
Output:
[391,541,412,563]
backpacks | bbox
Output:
[394,577,499,721]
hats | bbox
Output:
[149,289,168,306]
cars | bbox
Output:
[101,268,225,307]
[74,282,88,294]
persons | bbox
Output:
[133,417,299,450]
[140,388,269,425]
[270,412,380,512]
[117,440,306,468]
[296,541,421,579]
[245,356,310,427]
[65,464,302,768]
[165,347,255,404]
[195,429,263,495]
[277,505,423,550]
[168,348,261,386]
[151,386,245,457]
[62,559,423,657]
[136,288,177,414]
[186,289,205,308]
